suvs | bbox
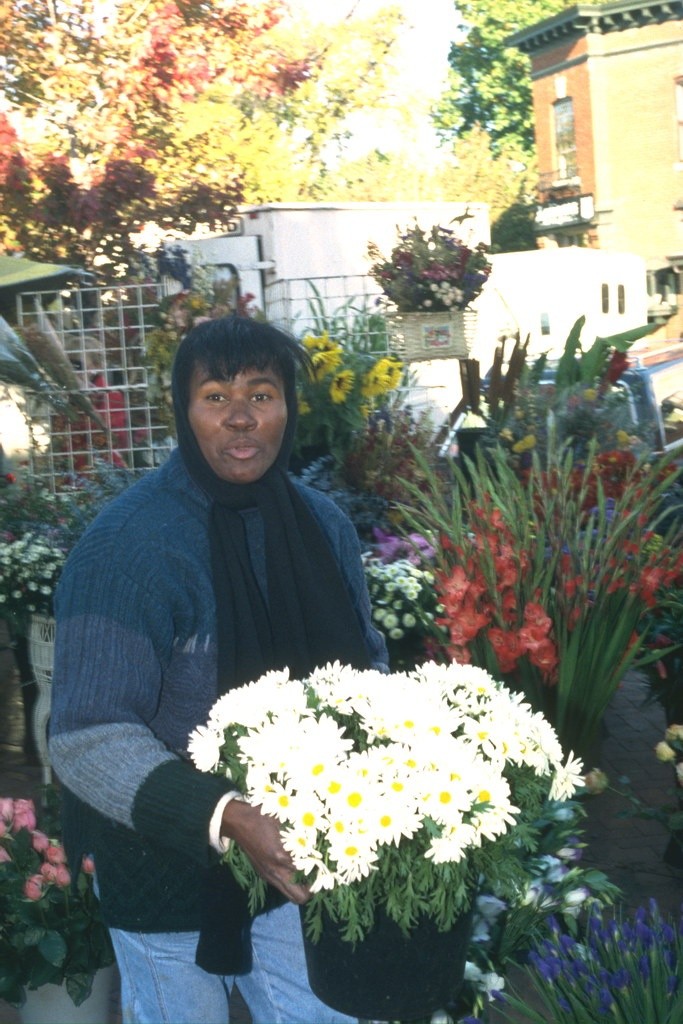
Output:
[0,257,140,519]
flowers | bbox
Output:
[0,207,683,1024]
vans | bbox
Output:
[423,347,683,555]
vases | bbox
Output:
[457,427,498,498]
[297,893,466,1021]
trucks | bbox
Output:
[156,201,490,344]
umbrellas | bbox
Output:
[0,253,73,327]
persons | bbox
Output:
[44,318,388,1024]
[51,336,124,476]
